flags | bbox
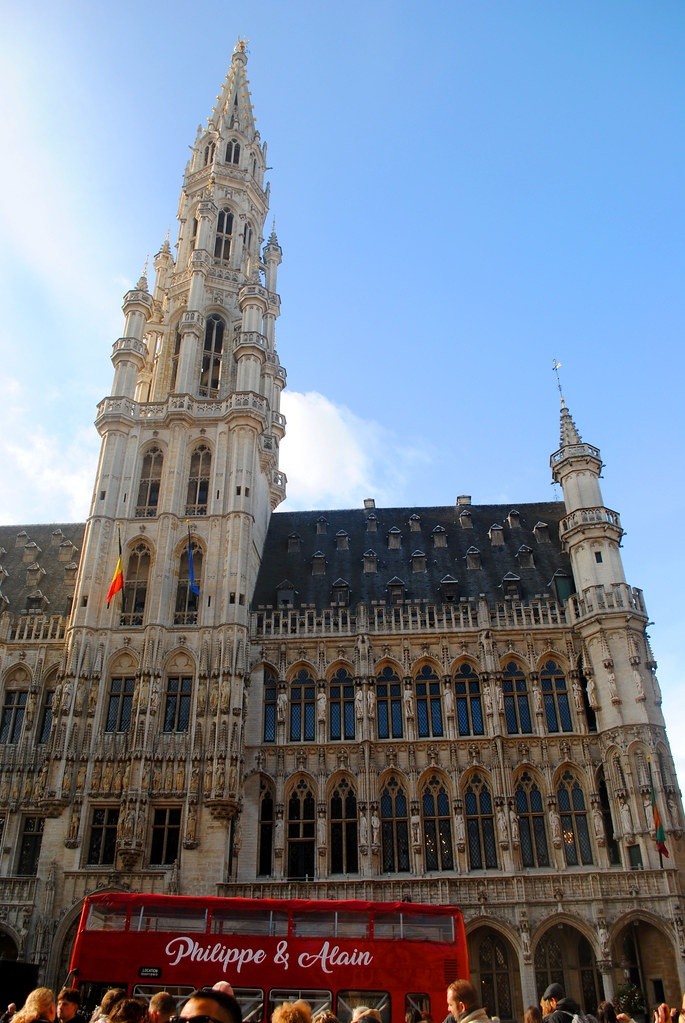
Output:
[650,771,669,858]
[188,531,200,597]
[106,538,124,609]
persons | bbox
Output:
[0,756,243,845]
[278,689,289,723]
[355,685,377,720]
[532,680,543,714]
[403,684,415,719]
[443,683,456,719]
[483,682,506,716]
[198,678,230,717]
[453,794,679,843]
[572,667,661,714]
[27,693,37,723]
[274,810,423,849]
[132,677,160,712]
[0,980,685,1023]
[52,678,98,717]
[317,687,327,723]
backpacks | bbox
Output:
[553,1007,597,1023]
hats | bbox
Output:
[543,983,563,1001]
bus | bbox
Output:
[61,891,470,1023]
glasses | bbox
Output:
[171,1015,222,1023]
